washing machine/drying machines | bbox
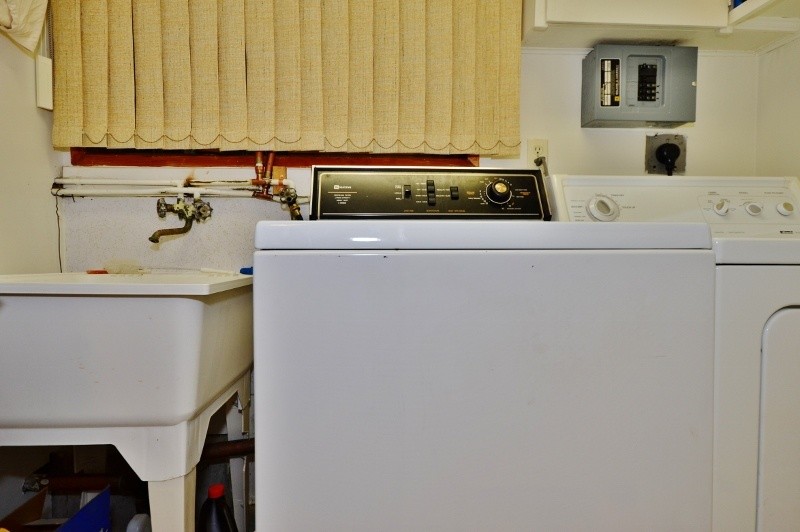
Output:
[550,172,794,528]
[244,162,717,528]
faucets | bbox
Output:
[146,197,214,245]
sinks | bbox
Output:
[0,274,254,429]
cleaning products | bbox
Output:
[197,484,239,531]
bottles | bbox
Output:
[195,484,240,532]
[126,505,151,532]
